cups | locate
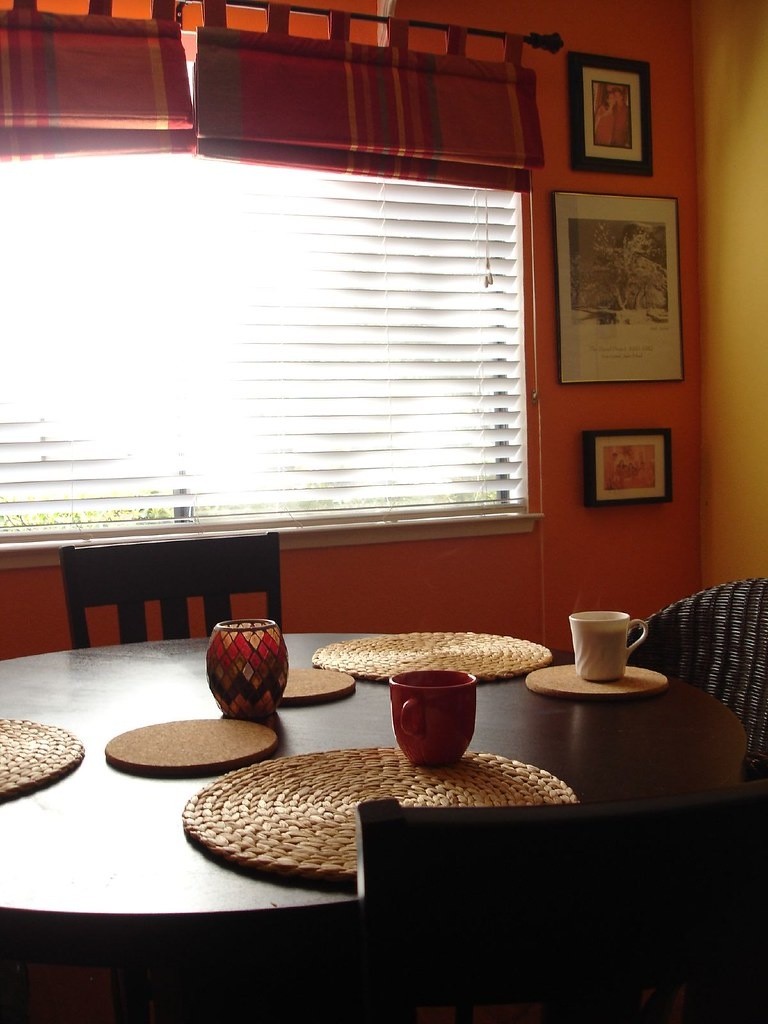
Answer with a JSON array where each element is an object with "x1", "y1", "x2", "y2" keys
[
  {"x1": 570, "y1": 612, "x2": 648, "y2": 682},
  {"x1": 388, "y1": 669, "x2": 478, "y2": 766}
]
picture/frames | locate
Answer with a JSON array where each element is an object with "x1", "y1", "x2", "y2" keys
[
  {"x1": 567, "y1": 50, "x2": 653, "y2": 176},
  {"x1": 582, "y1": 428, "x2": 673, "y2": 509},
  {"x1": 551, "y1": 190, "x2": 685, "y2": 384}
]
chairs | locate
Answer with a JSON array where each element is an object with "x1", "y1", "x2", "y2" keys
[
  {"x1": 356, "y1": 777, "x2": 768, "y2": 1024},
  {"x1": 625, "y1": 576, "x2": 768, "y2": 783},
  {"x1": 58, "y1": 531, "x2": 284, "y2": 650}
]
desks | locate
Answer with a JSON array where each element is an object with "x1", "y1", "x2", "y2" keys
[{"x1": 0, "y1": 631, "x2": 747, "y2": 1024}]
